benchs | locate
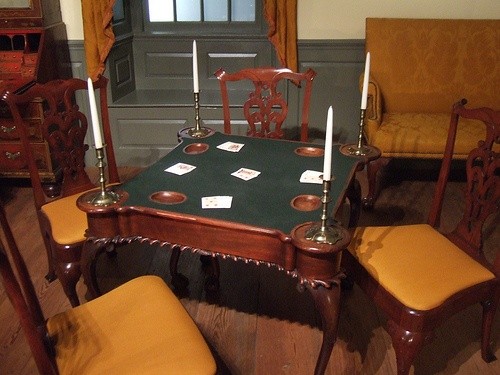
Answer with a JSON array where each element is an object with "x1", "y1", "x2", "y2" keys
[{"x1": 356, "y1": 17, "x2": 500, "y2": 208}]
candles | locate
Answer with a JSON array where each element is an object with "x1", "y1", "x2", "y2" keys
[
  {"x1": 359, "y1": 51, "x2": 370, "y2": 110},
  {"x1": 192, "y1": 39, "x2": 199, "y2": 92},
  {"x1": 87, "y1": 78, "x2": 102, "y2": 148},
  {"x1": 324, "y1": 104, "x2": 334, "y2": 180}
]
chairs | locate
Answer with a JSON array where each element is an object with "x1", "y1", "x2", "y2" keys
[
  {"x1": 0, "y1": 198, "x2": 228, "y2": 375},
  {"x1": 199, "y1": 67, "x2": 315, "y2": 265},
  {"x1": 314, "y1": 99, "x2": 500, "y2": 375},
  {"x1": 0, "y1": 74, "x2": 120, "y2": 308}
]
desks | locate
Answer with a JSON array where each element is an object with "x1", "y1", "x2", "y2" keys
[{"x1": 76, "y1": 128, "x2": 383, "y2": 375}]
